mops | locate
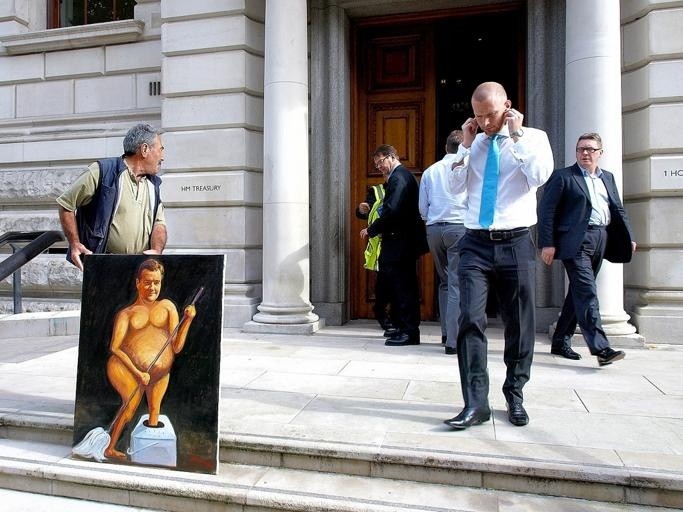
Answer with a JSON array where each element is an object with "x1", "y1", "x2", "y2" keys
[{"x1": 72, "y1": 285, "x2": 206, "y2": 463}]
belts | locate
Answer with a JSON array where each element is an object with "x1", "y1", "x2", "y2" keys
[
  {"x1": 427, "y1": 221, "x2": 464, "y2": 226},
  {"x1": 467, "y1": 228, "x2": 530, "y2": 242}
]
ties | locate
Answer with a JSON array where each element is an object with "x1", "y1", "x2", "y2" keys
[{"x1": 479, "y1": 134, "x2": 501, "y2": 230}]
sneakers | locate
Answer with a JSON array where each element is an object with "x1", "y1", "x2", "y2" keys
[{"x1": 441, "y1": 335, "x2": 458, "y2": 354}]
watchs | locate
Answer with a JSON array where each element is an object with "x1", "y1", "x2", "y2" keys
[{"x1": 510, "y1": 128, "x2": 524, "y2": 138}]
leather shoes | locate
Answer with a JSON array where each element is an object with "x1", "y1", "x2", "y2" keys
[
  {"x1": 384, "y1": 327, "x2": 420, "y2": 346},
  {"x1": 597, "y1": 347, "x2": 626, "y2": 366},
  {"x1": 444, "y1": 406, "x2": 491, "y2": 430},
  {"x1": 505, "y1": 401, "x2": 530, "y2": 427},
  {"x1": 550, "y1": 345, "x2": 582, "y2": 360}
]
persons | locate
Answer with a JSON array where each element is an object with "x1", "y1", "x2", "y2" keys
[
  {"x1": 537, "y1": 132, "x2": 636, "y2": 366},
  {"x1": 356, "y1": 172, "x2": 392, "y2": 329},
  {"x1": 360, "y1": 145, "x2": 430, "y2": 346},
  {"x1": 419, "y1": 129, "x2": 469, "y2": 354},
  {"x1": 443, "y1": 82, "x2": 554, "y2": 429},
  {"x1": 56, "y1": 124, "x2": 167, "y2": 271},
  {"x1": 104, "y1": 260, "x2": 196, "y2": 458}
]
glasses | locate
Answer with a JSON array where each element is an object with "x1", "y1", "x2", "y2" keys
[
  {"x1": 576, "y1": 147, "x2": 600, "y2": 153},
  {"x1": 374, "y1": 155, "x2": 390, "y2": 168}
]
